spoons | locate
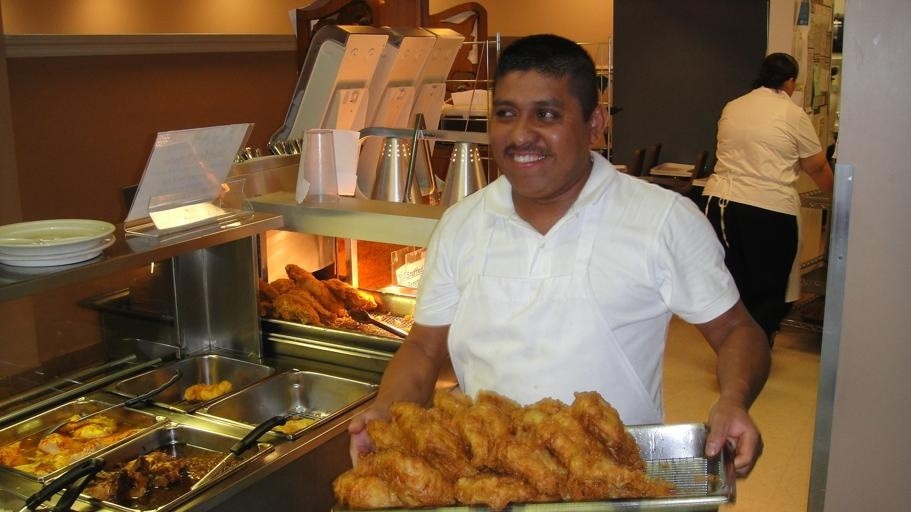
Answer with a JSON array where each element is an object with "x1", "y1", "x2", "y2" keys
[{"x1": 39, "y1": 370, "x2": 184, "y2": 444}]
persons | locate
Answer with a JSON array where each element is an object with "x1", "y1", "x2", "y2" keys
[
  {"x1": 701, "y1": 52, "x2": 835, "y2": 347},
  {"x1": 347, "y1": 34, "x2": 772, "y2": 480}
]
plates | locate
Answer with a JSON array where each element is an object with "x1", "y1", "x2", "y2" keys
[
  {"x1": 429, "y1": 423, "x2": 736, "y2": 511},
  {"x1": 650, "y1": 160, "x2": 697, "y2": 178},
  {"x1": 1, "y1": 221, "x2": 116, "y2": 269}
]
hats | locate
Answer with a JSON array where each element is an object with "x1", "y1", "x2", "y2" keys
[{"x1": 760, "y1": 53, "x2": 801, "y2": 82}]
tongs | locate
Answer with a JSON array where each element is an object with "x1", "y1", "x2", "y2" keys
[
  {"x1": 348, "y1": 305, "x2": 411, "y2": 340},
  {"x1": 402, "y1": 115, "x2": 440, "y2": 212}
]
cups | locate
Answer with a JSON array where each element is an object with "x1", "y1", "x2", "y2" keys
[{"x1": 295, "y1": 127, "x2": 339, "y2": 207}]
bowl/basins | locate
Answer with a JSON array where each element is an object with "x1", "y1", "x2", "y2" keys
[
  {"x1": 257, "y1": 286, "x2": 418, "y2": 353},
  {"x1": 195, "y1": 368, "x2": 381, "y2": 442},
  {"x1": 43, "y1": 420, "x2": 275, "y2": 512},
  {"x1": 0, "y1": 395, "x2": 170, "y2": 484},
  {"x1": 103, "y1": 353, "x2": 275, "y2": 415}
]
